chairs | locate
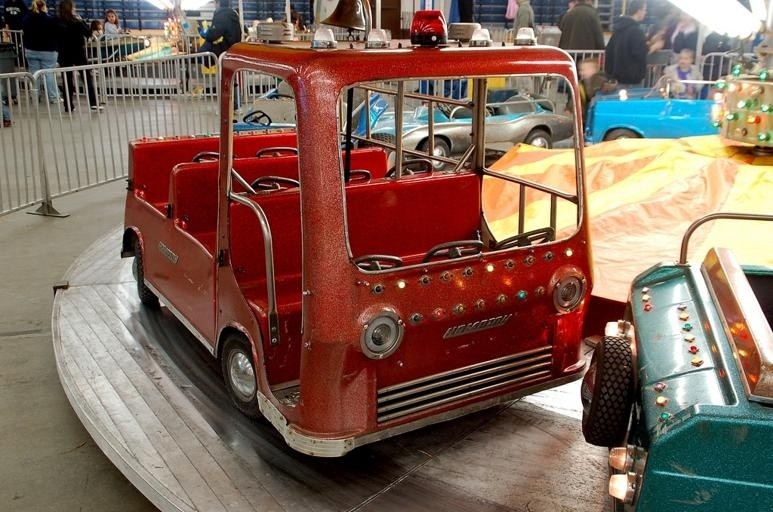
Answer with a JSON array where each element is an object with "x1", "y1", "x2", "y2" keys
[
  {"x1": 494, "y1": 95, "x2": 533, "y2": 116},
  {"x1": 441, "y1": 99, "x2": 489, "y2": 125}
]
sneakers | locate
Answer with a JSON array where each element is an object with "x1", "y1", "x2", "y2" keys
[
  {"x1": 51, "y1": 97, "x2": 65, "y2": 103},
  {"x1": 90, "y1": 104, "x2": 105, "y2": 111}
]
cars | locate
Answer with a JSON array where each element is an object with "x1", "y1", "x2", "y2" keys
[
  {"x1": 227, "y1": 81, "x2": 306, "y2": 129},
  {"x1": 586, "y1": 76, "x2": 716, "y2": 142},
  {"x1": 368, "y1": 89, "x2": 575, "y2": 172}
]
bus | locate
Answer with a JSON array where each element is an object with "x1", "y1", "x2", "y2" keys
[{"x1": 120, "y1": 8, "x2": 598, "y2": 462}]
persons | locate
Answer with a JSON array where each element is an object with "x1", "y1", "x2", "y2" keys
[
  {"x1": 102, "y1": 8, "x2": 124, "y2": 37},
  {"x1": 87, "y1": 20, "x2": 105, "y2": 42},
  {"x1": 662, "y1": 46, "x2": 710, "y2": 101},
  {"x1": 564, "y1": 60, "x2": 612, "y2": 126},
  {"x1": 505, "y1": 0, "x2": 772, "y2": 87},
  {"x1": 21, "y1": 0, "x2": 60, "y2": 106},
  {"x1": 193, "y1": 0, "x2": 241, "y2": 116},
  {"x1": 3, "y1": 0, "x2": 29, "y2": 68},
  {"x1": 0, "y1": 25, "x2": 16, "y2": 129},
  {"x1": 53, "y1": 1, "x2": 102, "y2": 112},
  {"x1": 284, "y1": 4, "x2": 302, "y2": 22}
]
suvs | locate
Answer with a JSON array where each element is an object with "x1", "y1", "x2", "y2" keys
[{"x1": 581, "y1": 206, "x2": 772, "y2": 510}]
